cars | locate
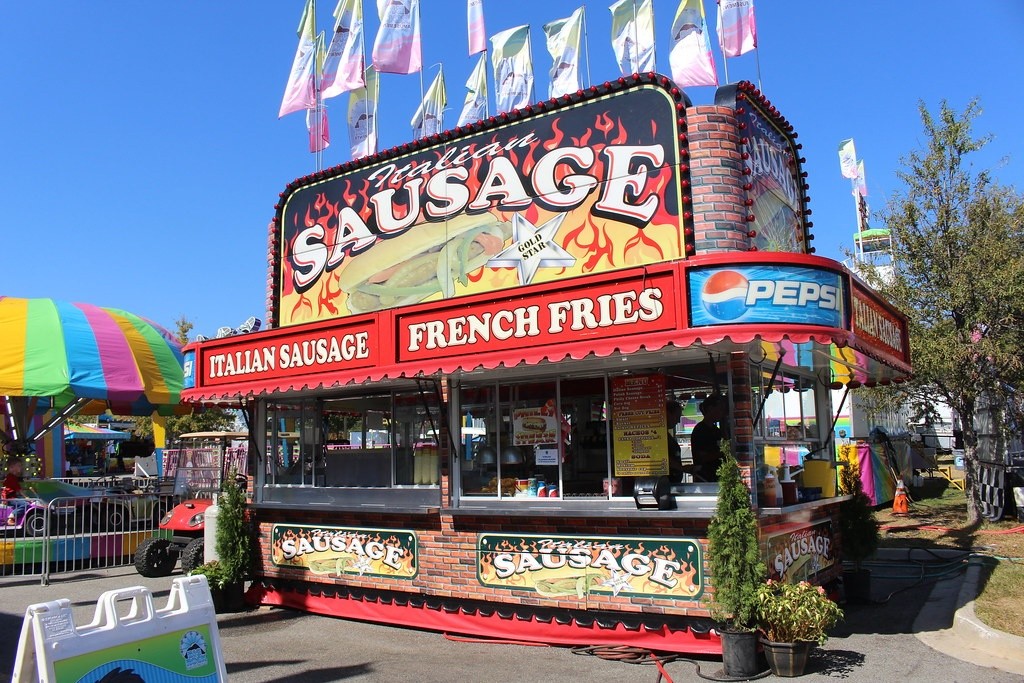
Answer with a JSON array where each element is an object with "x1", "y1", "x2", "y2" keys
[
  {"x1": 87, "y1": 482, "x2": 166, "y2": 532},
  {"x1": 0, "y1": 486, "x2": 55, "y2": 537}
]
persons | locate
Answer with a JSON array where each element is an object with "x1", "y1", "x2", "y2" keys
[
  {"x1": 666, "y1": 400, "x2": 684, "y2": 484},
  {"x1": 691, "y1": 392, "x2": 730, "y2": 482},
  {"x1": 1, "y1": 458, "x2": 31, "y2": 526}
]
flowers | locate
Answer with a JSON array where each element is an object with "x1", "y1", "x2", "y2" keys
[{"x1": 756, "y1": 574, "x2": 845, "y2": 644}]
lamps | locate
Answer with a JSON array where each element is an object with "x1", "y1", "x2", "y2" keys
[{"x1": 476, "y1": 384, "x2": 527, "y2": 468}]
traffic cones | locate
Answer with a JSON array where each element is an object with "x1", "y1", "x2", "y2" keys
[{"x1": 888, "y1": 472, "x2": 910, "y2": 518}]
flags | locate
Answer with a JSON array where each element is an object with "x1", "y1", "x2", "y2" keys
[
  {"x1": 277, "y1": 0, "x2": 758, "y2": 162},
  {"x1": 838, "y1": 138, "x2": 866, "y2": 196}
]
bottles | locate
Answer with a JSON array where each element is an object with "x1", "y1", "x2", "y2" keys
[
  {"x1": 765, "y1": 464, "x2": 776, "y2": 507},
  {"x1": 770, "y1": 420, "x2": 785, "y2": 437}
]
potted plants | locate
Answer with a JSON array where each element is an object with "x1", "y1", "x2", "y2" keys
[
  {"x1": 701, "y1": 434, "x2": 771, "y2": 677},
  {"x1": 835, "y1": 440, "x2": 881, "y2": 606},
  {"x1": 187, "y1": 461, "x2": 249, "y2": 613}
]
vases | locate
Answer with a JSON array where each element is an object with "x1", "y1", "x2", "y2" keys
[{"x1": 761, "y1": 635, "x2": 812, "y2": 676}]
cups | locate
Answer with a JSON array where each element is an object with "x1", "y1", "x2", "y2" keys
[
  {"x1": 528, "y1": 478, "x2": 537, "y2": 496},
  {"x1": 538, "y1": 481, "x2": 546, "y2": 497},
  {"x1": 549, "y1": 485, "x2": 557, "y2": 497}
]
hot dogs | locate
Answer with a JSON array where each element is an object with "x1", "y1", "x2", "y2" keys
[
  {"x1": 522, "y1": 417, "x2": 547, "y2": 432},
  {"x1": 788, "y1": 554, "x2": 812, "y2": 582}
]
